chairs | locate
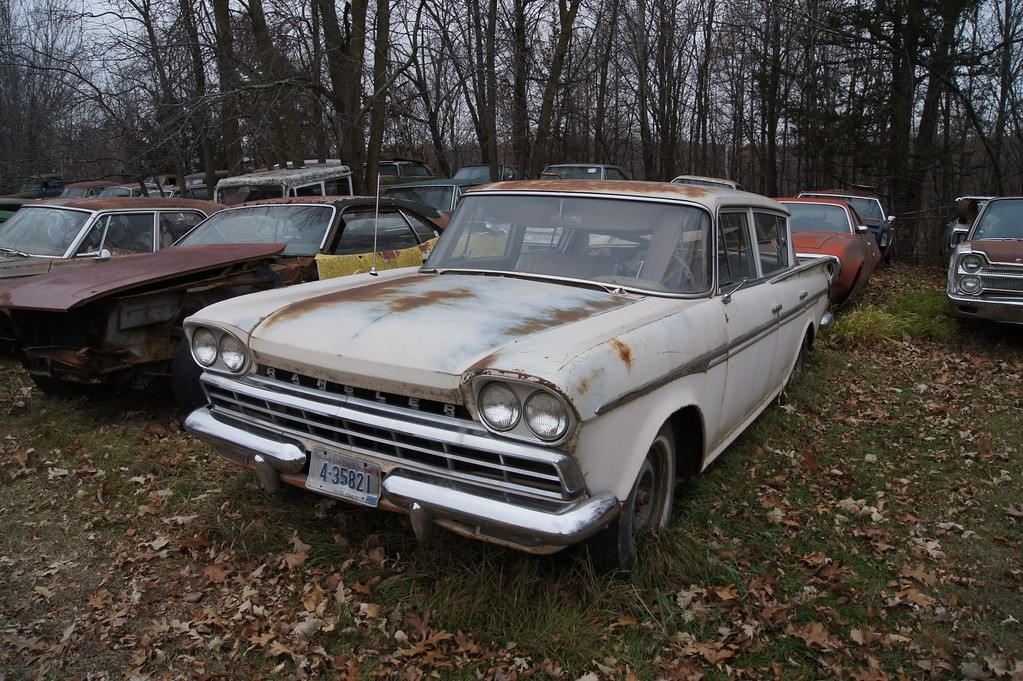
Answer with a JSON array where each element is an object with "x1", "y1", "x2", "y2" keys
[
  {"x1": 291, "y1": 220, "x2": 420, "y2": 254},
  {"x1": 521, "y1": 237, "x2": 765, "y2": 294},
  {"x1": 92, "y1": 222, "x2": 187, "y2": 251}
]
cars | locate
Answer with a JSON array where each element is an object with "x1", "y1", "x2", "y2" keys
[
  {"x1": 1, "y1": 198, "x2": 245, "y2": 345},
  {"x1": 771, "y1": 198, "x2": 881, "y2": 307},
  {"x1": 942, "y1": 197, "x2": 995, "y2": 265},
  {"x1": 793, "y1": 191, "x2": 895, "y2": 266},
  {"x1": 1, "y1": 157, "x2": 747, "y2": 247},
  {"x1": 1, "y1": 196, "x2": 530, "y2": 414},
  {"x1": 946, "y1": 196, "x2": 1022, "y2": 330}
]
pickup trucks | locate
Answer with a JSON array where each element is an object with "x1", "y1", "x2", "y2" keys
[{"x1": 182, "y1": 177, "x2": 842, "y2": 581}]
